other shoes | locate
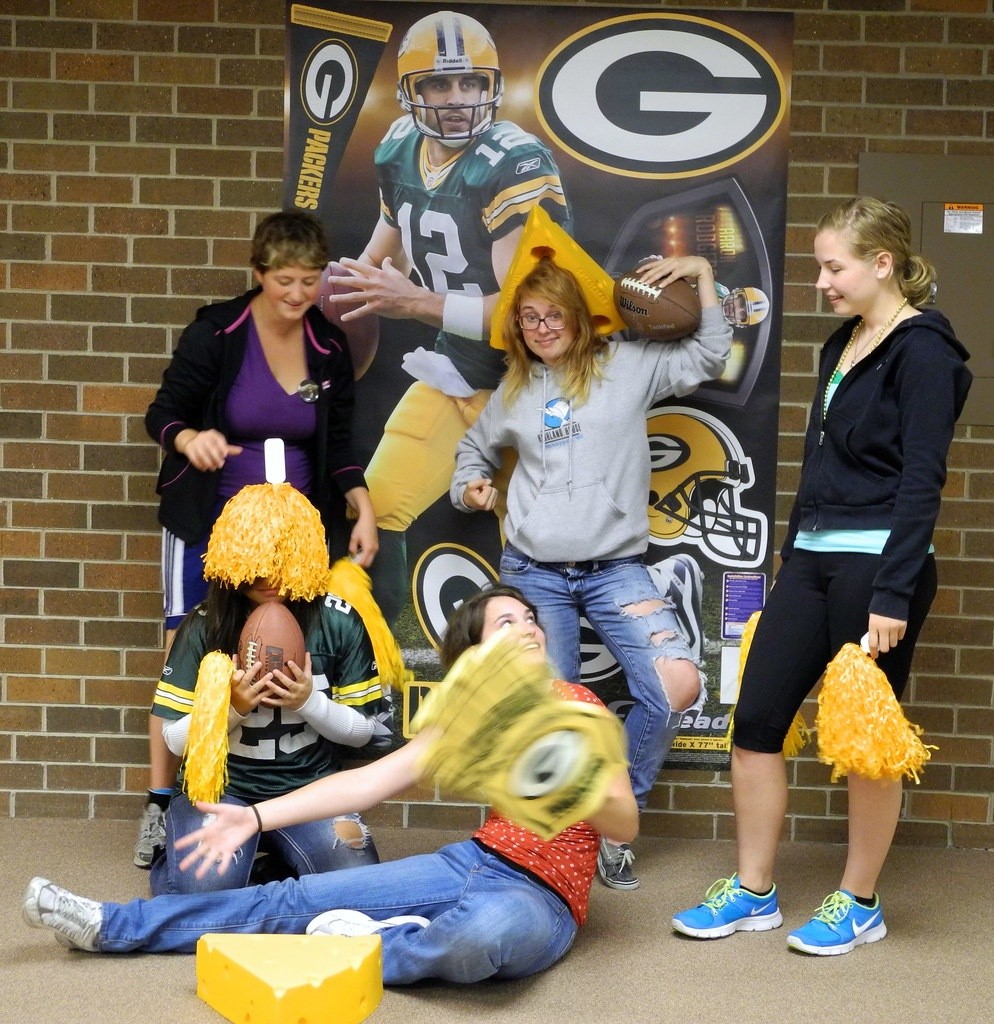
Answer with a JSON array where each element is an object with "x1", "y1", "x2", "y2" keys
[{"x1": 652, "y1": 554, "x2": 710, "y2": 670}]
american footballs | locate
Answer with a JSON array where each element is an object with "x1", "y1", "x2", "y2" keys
[
  {"x1": 237, "y1": 598, "x2": 305, "y2": 704},
  {"x1": 316, "y1": 264, "x2": 380, "y2": 356},
  {"x1": 612, "y1": 270, "x2": 702, "y2": 343}
]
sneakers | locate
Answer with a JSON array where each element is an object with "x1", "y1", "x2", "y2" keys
[
  {"x1": 787, "y1": 890, "x2": 887, "y2": 955},
  {"x1": 22, "y1": 877, "x2": 102, "y2": 951},
  {"x1": 133, "y1": 803, "x2": 167, "y2": 867},
  {"x1": 673, "y1": 871, "x2": 783, "y2": 939},
  {"x1": 306, "y1": 909, "x2": 431, "y2": 938},
  {"x1": 597, "y1": 840, "x2": 639, "y2": 890}
]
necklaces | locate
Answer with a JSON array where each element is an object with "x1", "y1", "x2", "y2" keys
[{"x1": 822, "y1": 296, "x2": 909, "y2": 420}]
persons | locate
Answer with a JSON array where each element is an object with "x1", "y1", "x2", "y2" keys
[
  {"x1": 133, "y1": 209, "x2": 381, "y2": 867},
  {"x1": 595, "y1": 250, "x2": 772, "y2": 345},
  {"x1": 447, "y1": 252, "x2": 736, "y2": 888},
  {"x1": 21, "y1": 579, "x2": 639, "y2": 986},
  {"x1": 328, "y1": 10, "x2": 710, "y2": 667},
  {"x1": 672, "y1": 195, "x2": 972, "y2": 953},
  {"x1": 146, "y1": 479, "x2": 393, "y2": 901}
]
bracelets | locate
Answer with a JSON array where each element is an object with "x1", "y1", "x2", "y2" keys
[
  {"x1": 441, "y1": 292, "x2": 485, "y2": 342},
  {"x1": 250, "y1": 804, "x2": 263, "y2": 834}
]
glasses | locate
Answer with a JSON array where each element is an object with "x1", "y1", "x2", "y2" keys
[{"x1": 516, "y1": 313, "x2": 567, "y2": 329}]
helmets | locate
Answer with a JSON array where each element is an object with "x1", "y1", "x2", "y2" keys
[
  {"x1": 396, "y1": 11, "x2": 504, "y2": 127},
  {"x1": 721, "y1": 286, "x2": 770, "y2": 326}
]
